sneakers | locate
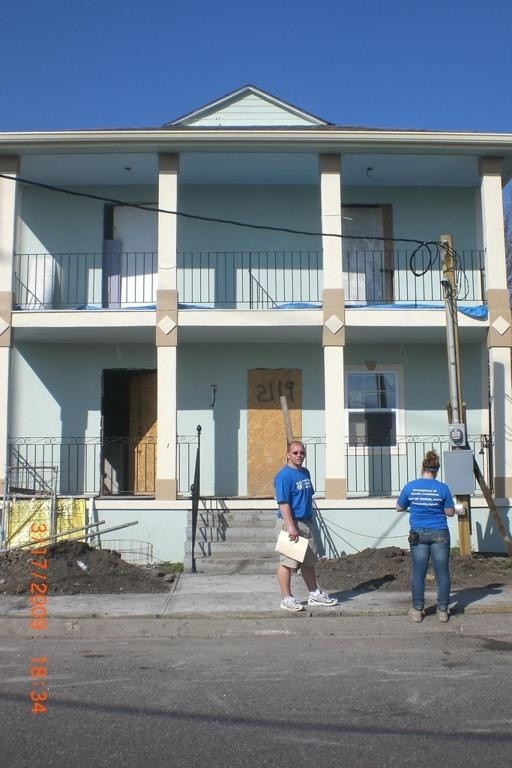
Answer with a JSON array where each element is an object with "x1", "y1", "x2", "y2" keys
[
  {"x1": 410, "y1": 607, "x2": 423, "y2": 622},
  {"x1": 307, "y1": 592, "x2": 338, "y2": 606},
  {"x1": 280, "y1": 596, "x2": 305, "y2": 612},
  {"x1": 436, "y1": 609, "x2": 449, "y2": 623}
]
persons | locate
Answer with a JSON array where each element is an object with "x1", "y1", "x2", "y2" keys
[
  {"x1": 273, "y1": 441, "x2": 338, "y2": 612},
  {"x1": 395, "y1": 450, "x2": 455, "y2": 623}
]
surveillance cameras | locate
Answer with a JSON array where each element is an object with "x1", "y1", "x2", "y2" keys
[{"x1": 440, "y1": 275, "x2": 448, "y2": 286}]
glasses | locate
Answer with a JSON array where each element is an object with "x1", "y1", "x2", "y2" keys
[{"x1": 292, "y1": 450, "x2": 305, "y2": 455}]
